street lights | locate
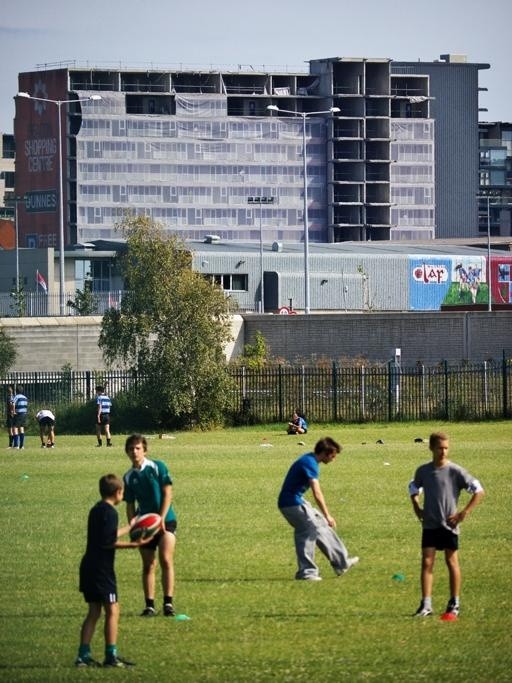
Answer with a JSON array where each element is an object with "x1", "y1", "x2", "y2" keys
[
  {"x1": 247, "y1": 194, "x2": 274, "y2": 314},
  {"x1": 475, "y1": 189, "x2": 501, "y2": 311},
  {"x1": 266, "y1": 103, "x2": 342, "y2": 314},
  {"x1": 12, "y1": 91, "x2": 101, "y2": 313},
  {"x1": 3, "y1": 193, "x2": 30, "y2": 316}
]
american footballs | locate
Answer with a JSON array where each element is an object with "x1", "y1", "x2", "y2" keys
[{"x1": 130, "y1": 512, "x2": 162, "y2": 542}]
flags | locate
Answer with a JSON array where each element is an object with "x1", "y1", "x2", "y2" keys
[{"x1": 32, "y1": 270, "x2": 48, "y2": 295}]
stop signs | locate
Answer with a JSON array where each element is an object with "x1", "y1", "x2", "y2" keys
[{"x1": 278, "y1": 306, "x2": 289, "y2": 315}]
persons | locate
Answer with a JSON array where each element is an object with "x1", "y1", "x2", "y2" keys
[
  {"x1": 35, "y1": 409, "x2": 57, "y2": 448},
  {"x1": 13, "y1": 384, "x2": 29, "y2": 450},
  {"x1": 277, "y1": 435, "x2": 362, "y2": 582},
  {"x1": 285, "y1": 412, "x2": 309, "y2": 434},
  {"x1": 5, "y1": 394, "x2": 16, "y2": 448},
  {"x1": 119, "y1": 431, "x2": 179, "y2": 618},
  {"x1": 406, "y1": 431, "x2": 486, "y2": 622},
  {"x1": 94, "y1": 385, "x2": 113, "y2": 445},
  {"x1": 74, "y1": 471, "x2": 155, "y2": 668}
]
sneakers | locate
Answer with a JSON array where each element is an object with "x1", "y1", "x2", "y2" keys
[
  {"x1": 51, "y1": 445, "x2": 54, "y2": 449},
  {"x1": 6, "y1": 447, "x2": 12, "y2": 449},
  {"x1": 335, "y1": 556, "x2": 359, "y2": 577},
  {"x1": 444, "y1": 607, "x2": 459, "y2": 616},
  {"x1": 20, "y1": 446, "x2": 24, "y2": 450},
  {"x1": 106, "y1": 443, "x2": 112, "y2": 447},
  {"x1": 103, "y1": 656, "x2": 136, "y2": 669},
  {"x1": 164, "y1": 603, "x2": 174, "y2": 615},
  {"x1": 75, "y1": 656, "x2": 101, "y2": 669},
  {"x1": 13, "y1": 447, "x2": 19, "y2": 449},
  {"x1": 144, "y1": 606, "x2": 155, "y2": 617},
  {"x1": 412, "y1": 608, "x2": 434, "y2": 617},
  {"x1": 41, "y1": 445, "x2": 46, "y2": 448},
  {"x1": 95, "y1": 444, "x2": 102, "y2": 447},
  {"x1": 298, "y1": 575, "x2": 322, "y2": 581}
]
